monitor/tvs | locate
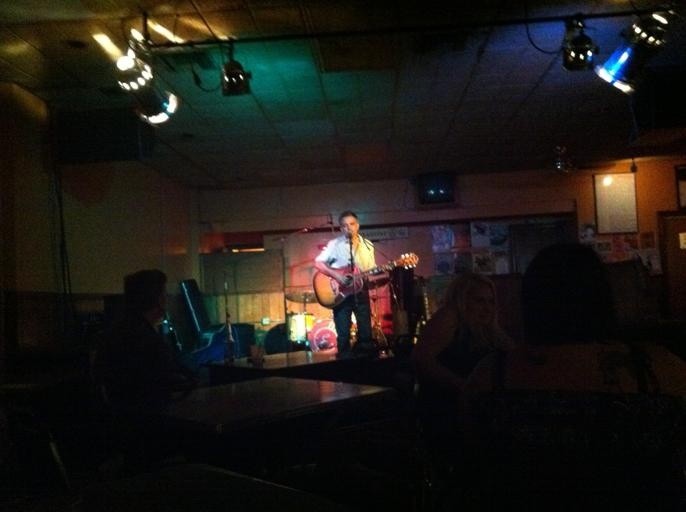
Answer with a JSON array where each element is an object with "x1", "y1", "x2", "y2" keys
[{"x1": 415, "y1": 169, "x2": 459, "y2": 209}]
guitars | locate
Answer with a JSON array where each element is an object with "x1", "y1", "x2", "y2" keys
[{"x1": 313, "y1": 253, "x2": 420, "y2": 309}]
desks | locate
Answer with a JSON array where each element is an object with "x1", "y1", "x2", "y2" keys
[
  {"x1": 229, "y1": 348, "x2": 365, "y2": 372},
  {"x1": 140, "y1": 375, "x2": 403, "y2": 429}
]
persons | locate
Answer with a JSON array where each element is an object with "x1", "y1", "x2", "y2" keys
[
  {"x1": 313, "y1": 209, "x2": 390, "y2": 359},
  {"x1": 454, "y1": 240, "x2": 686, "y2": 439},
  {"x1": 414, "y1": 270, "x2": 515, "y2": 457},
  {"x1": 92, "y1": 267, "x2": 196, "y2": 399}
]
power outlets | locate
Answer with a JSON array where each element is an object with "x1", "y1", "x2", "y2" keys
[{"x1": 216, "y1": 37, "x2": 254, "y2": 100}]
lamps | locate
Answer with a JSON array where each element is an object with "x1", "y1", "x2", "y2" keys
[
  {"x1": 594, "y1": 4, "x2": 678, "y2": 99},
  {"x1": 115, "y1": 36, "x2": 180, "y2": 127},
  {"x1": 562, "y1": 14, "x2": 600, "y2": 71}
]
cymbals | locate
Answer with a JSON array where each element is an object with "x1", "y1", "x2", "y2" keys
[
  {"x1": 369, "y1": 294, "x2": 386, "y2": 301},
  {"x1": 366, "y1": 278, "x2": 390, "y2": 290},
  {"x1": 284, "y1": 289, "x2": 317, "y2": 303}
]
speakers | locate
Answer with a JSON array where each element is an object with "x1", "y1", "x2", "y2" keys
[{"x1": 55, "y1": 107, "x2": 151, "y2": 164}]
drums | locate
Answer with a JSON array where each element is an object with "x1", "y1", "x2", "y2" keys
[
  {"x1": 307, "y1": 317, "x2": 339, "y2": 353},
  {"x1": 286, "y1": 312, "x2": 314, "y2": 343}
]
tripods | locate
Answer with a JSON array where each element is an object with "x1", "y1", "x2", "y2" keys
[{"x1": 371, "y1": 287, "x2": 395, "y2": 357}]
